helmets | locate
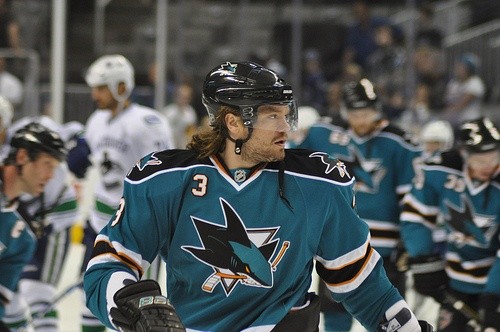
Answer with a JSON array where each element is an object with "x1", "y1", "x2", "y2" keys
[
  {"x1": 84, "y1": 54, "x2": 135, "y2": 100},
  {"x1": 202, "y1": 60, "x2": 294, "y2": 126},
  {"x1": 341, "y1": 79, "x2": 379, "y2": 113},
  {"x1": 454, "y1": 115, "x2": 500, "y2": 152},
  {"x1": 10, "y1": 122, "x2": 68, "y2": 160},
  {"x1": 421, "y1": 121, "x2": 454, "y2": 149}
]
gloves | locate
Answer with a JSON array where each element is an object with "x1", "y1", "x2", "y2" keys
[
  {"x1": 407, "y1": 252, "x2": 450, "y2": 303},
  {"x1": 64, "y1": 134, "x2": 110, "y2": 178},
  {"x1": 110, "y1": 279, "x2": 186, "y2": 332}
]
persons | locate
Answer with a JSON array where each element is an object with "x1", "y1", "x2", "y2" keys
[
  {"x1": 284, "y1": 77, "x2": 500, "y2": 332},
  {"x1": 83, "y1": 54, "x2": 177, "y2": 332},
  {"x1": 163, "y1": 83, "x2": 197, "y2": 145},
  {"x1": 84, "y1": 61, "x2": 433, "y2": 332},
  {"x1": 303, "y1": 0, "x2": 484, "y2": 159},
  {"x1": 0, "y1": 71, "x2": 85, "y2": 332}
]
